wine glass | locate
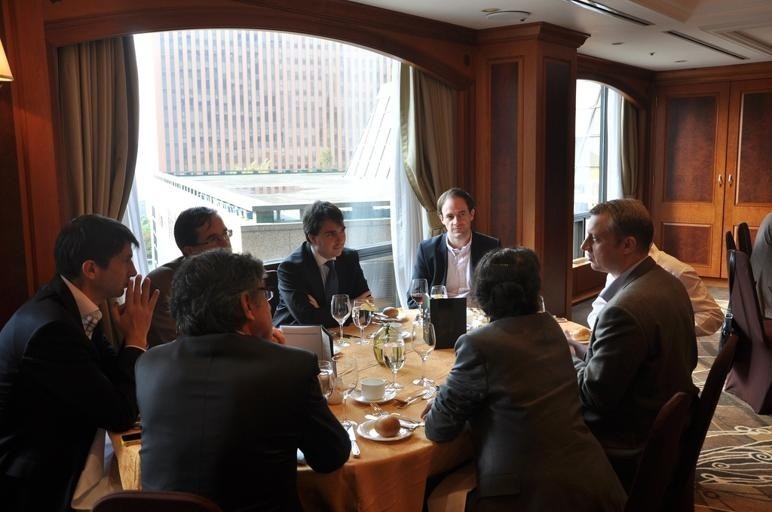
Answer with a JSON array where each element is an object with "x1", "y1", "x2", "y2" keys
[{"x1": 317, "y1": 278, "x2": 449, "y2": 404}]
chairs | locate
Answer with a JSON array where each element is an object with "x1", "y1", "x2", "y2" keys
[
  {"x1": 734, "y1": 222, "x2": 753, "y2": 259},
  {"x1": 720, "y1": 249, "x2": 771, "y2": 415},
  {"x1": 723, "y1": 229, "x2": 734, "y2": 274},
  {"x1": 92, "y1": 488, "x2": 219, "y2": 512},
  {"x1": 622, "y1": 387, "x2": 695, "y2": 511},
  {"x1": 693, "y1": 330, "x2": 736, "y2": 463}
]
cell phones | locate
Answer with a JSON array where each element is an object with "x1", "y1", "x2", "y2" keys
[{"x1": 119, "y1": 432, "x2": 142, "y2": 447}]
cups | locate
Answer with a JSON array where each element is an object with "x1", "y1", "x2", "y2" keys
[{"x1": 538, "y1": 294, "x2": 545, "y2": 313}]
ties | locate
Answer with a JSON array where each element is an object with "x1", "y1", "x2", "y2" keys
[{"x1": 325, "y1": 260, "x2": 339, "y2": 305}]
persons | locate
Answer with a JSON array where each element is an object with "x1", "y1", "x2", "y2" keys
[
  {"x1": 749, "y1": 212, "x2": 772, "y2": 318},
  {"x1": 142, "y1": 207, "x2": 230, "y2": 347},
  {"x1": 568, "y1": 198, "x2": 698, "y2": 446},
  {"x1": 273, "y1": 202, "x2": 375, "y2": 328},
  {"x1": 587, "y1": 244, "x2": 725, "y2": 338},
  {"x1": 408, "y1": 186, "x2": 500, "y2": 309},
  {"x1": 0, "y1": 213, "x2": 161, "y2": 512},
  {"x1": 134, "y1": 248, "x2": 351, "y2": 512},
  {"x1": 424, "y1": 246, "x2": 630, "y2": 512}
]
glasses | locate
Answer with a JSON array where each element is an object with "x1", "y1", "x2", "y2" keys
[
  {"x1": 232, "y1": 284, "x2": 274, "y2": 304},
  {"x1": 189, "y1": 229, "x2": 233, "y2": 249}
]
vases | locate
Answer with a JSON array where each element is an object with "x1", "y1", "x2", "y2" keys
[{"x1": 372, "y1": 323, "x2": 406, "y2": 369}]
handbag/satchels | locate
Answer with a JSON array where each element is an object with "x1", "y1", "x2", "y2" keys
[{"x1": 718, "y1": 321, "x2": 732, "y2": 352}]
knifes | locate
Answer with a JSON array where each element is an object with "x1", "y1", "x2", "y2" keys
[{"x1": 348, "y1": 424, "x2": 361, "y2": 458}]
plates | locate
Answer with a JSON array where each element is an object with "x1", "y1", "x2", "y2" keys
[
  {"x1": 357, "y1": 419, "x2": 412, "y2": 442},
  {"x1": 572, "y1": 328, "x2": 591, "y2": 345}
]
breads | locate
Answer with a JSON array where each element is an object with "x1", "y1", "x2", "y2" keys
[
  {"x1": 574, "y1": 328, "x2": 590, "y2": 341},
  {"x1": 383, "y1": 307, "x2": 398, "y2": 317},
  {"x1": 374, "y1": 415, "x2": 400, "y2": 438}
]
